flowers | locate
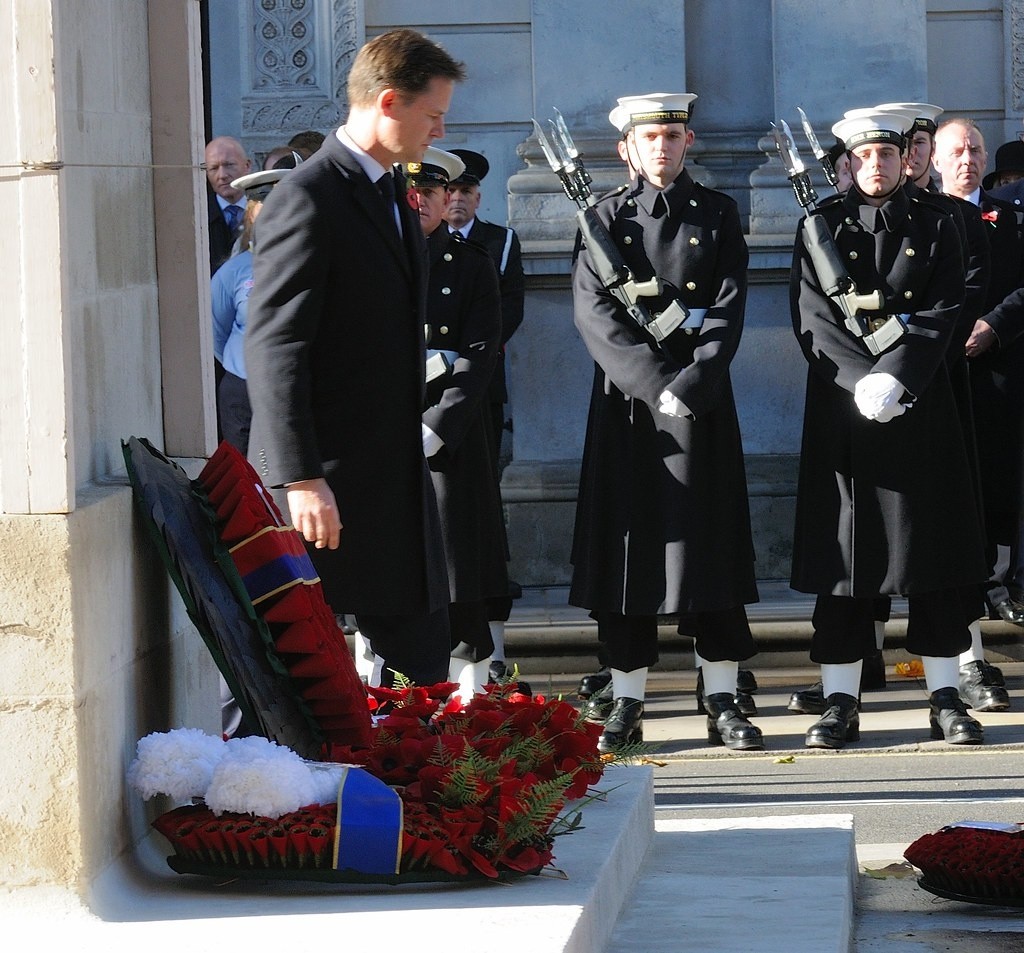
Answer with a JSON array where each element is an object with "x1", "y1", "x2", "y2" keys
[
  {"x1": 980, "y1": 210, "x2": 999, "y2": 229},
  {"x1": 403, "y1": 176, "x2": 417, "y2": 211},
  {"x1": 904, "y1": 819, "x2": 1024, "y2": 909},
  {"x1": 200, "y1": 432, "x2": 374, "y2": 747},
  {"x1": 127, "y1": 672, "x2": 669, "y2": 892}
]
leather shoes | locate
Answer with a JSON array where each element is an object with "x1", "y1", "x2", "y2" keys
[
  {"x1": 696, "y1": 672, "x2": 757, "y2": 714},
  {"x1": 578, "y1": 667, "x2": 611, "y2": 698},
  {"x1": 586, "y1": 681, "x2": 613, "y2": 721},
  {"x1": 958, "y1": 660, "x2": 1010, "y2": 711},
  {"x1": 596, "y1": 696, "x2": 645, "y2": 752},
  {"x1": 804, "y1": 703, "x2": 860, "y2": 747},
  {"x1": 928, "y1": 686, "x2": 984, "y2": 742},
  {"x1": 989, "y1": 598, "x2": 1023, "y2": 623},
  {"x1": 983, "y1": 664, "x2": 1005, "y2": 687},
  {"x1": 488, "y1": 662, "x2": 532, "y2": 699},
  {"x1": 706, "y1": 704, "x2": 765, "y2": 750},
  {"x1": 737, "y1": 670, "x2": 757, "y2": 693},
  {"x1": 787, "y1": 680, "x2": 827, "y2": 714}
]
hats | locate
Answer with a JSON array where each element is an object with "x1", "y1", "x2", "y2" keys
[
  {"x1": 408, "y1": 144, "x2": 467, "y2": 190},
  {"x1": 230, "y1": 168, "x2": 296, "y2": 197},
  {"x1": 443, "y1": 148, "x2": 491, "y2": 185},
  {"x1": 606, "y1": 92, "x2": 945, "y2": 150}
]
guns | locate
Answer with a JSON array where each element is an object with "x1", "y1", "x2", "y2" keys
[
  {"x1": 530, "y1": 103, "x2": 691, "y2": 353},
  {"x1": 770, "y1": 107, "x2": 908, "y2": 358}
]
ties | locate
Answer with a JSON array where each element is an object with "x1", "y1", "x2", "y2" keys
[
  {"x1": 224, "y1": 204, "x2": 239, "y2": 236},
  {"x1": 379, "y1": 173, "x2": 398, "y2": 238}
]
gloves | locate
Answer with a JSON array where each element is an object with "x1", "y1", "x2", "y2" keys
[
  {"x1": 658, "y1": 390, "x2": 692, "y2": 417},
  {"x1": 421, "y1": 423, "x2": 444, "y2": 457},
  {"x1": 854, "y1": 373, "x2": 907, "y2": 423}
]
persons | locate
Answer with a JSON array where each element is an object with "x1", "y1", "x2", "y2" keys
[
  {"x1": 243, "y1": 28, "x2": 469, "y2": 710},
  {"x1": 207, "y1": 90, "x2": 1024, "y2": 750}
]
